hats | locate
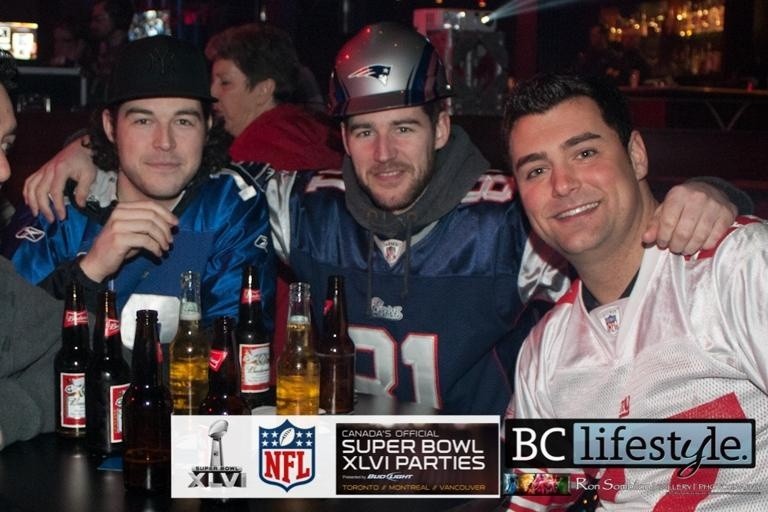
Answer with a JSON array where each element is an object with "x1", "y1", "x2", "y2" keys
[{"x1": 109, "y1": 35, "x2": 210, "y2": 101}]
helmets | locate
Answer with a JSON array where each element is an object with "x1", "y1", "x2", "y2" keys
[{"x1": 329, "y1": 20, "x2": 454, "y2": 117}]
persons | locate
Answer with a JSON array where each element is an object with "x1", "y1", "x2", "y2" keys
[
  {"x1": 21, "y1": 21, "x2": 755, "y2": 420},
  {"x1": 500, "y1": 72, "x2": 764, "y2": 512},
  {"x1": 206, "y1": 22, "x2": 344, "y2": 171},
  {"x1": 0, "y1": 47, "x2": 97, "y2": 448},
  {"x1": 9, "y1": 36, "x2": 276, "y2": 383}
]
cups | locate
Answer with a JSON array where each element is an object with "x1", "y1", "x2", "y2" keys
[{"x1": 629, "y1": 70, "x2": 641, "y2": 88}]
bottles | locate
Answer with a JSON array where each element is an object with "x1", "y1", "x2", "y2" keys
[
  {"x1": 669, "y1": 42, "x2": 720, "y2": 75},
  {"x1": 235, "y1": 264, "x2": 275, "y2": 408},
  {"x1": 198, "y1": 315, "x2": 251, "y2": 415},
  {"x1": 609, "y1": 0, "x2": 725, "y2": 43},
  {"x1": 122, "y1": 311, "x2": 174, "y2": 490},
  {"x1": 86, "y1": 291, "x2": 131, "y2": 458},
  {"x1": 53, "y1": 279, "x2": 93, "y2": 437},
  {"x1": 315, "y1": 274, "x2": 355, "y2": 415},
  {"x1": 276, "y1": 282, "x2": 321, "y2": 415},
  {"x1": 168, "y1": 271, "x2": 211, "y2": 415}
]
